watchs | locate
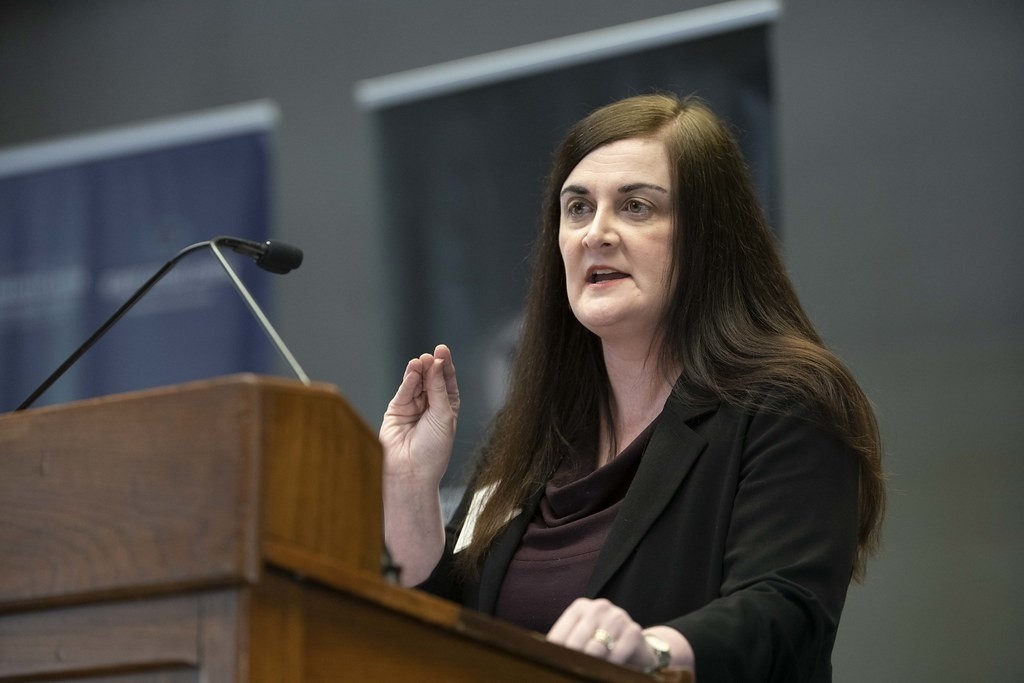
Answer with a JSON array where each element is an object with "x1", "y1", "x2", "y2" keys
[{"x1": 641, "y1": 630, "x2": 672, "y2": 675}]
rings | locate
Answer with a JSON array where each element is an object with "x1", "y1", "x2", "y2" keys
[{"x1": 592, "y1": 628, "x2": 616, "y2": 652}]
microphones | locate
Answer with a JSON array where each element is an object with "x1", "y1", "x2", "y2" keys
[{"x1": 12, "y1": 239, "x2": 312, "y2": 416}]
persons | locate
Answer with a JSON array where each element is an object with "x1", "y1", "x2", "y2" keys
[{"x1": 377, "y1": 95, "x2": 891, "y2": 683}]
